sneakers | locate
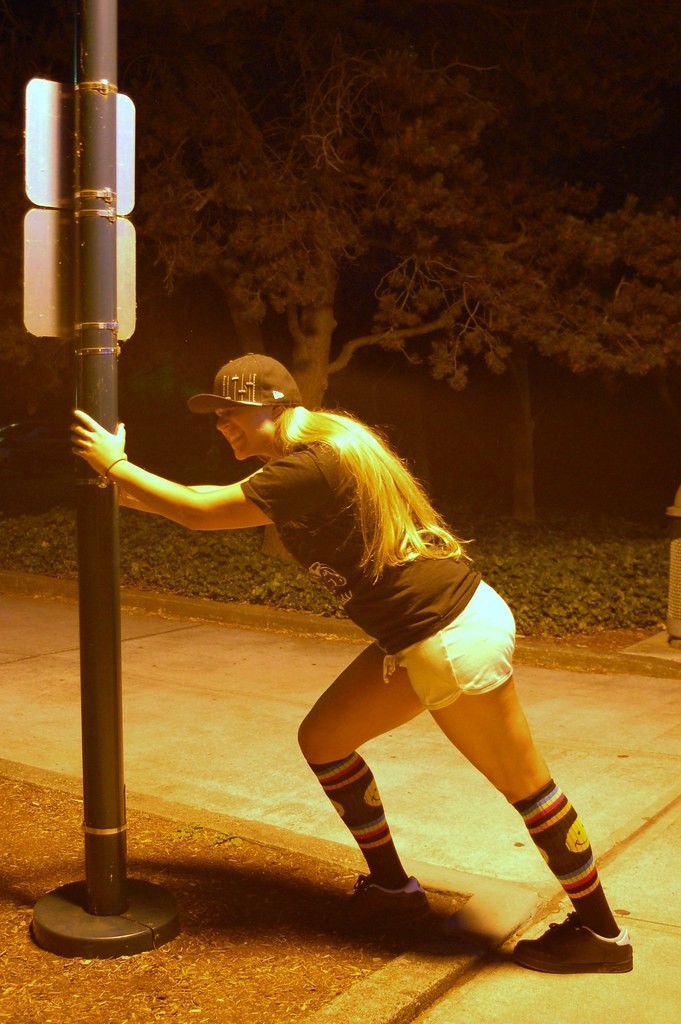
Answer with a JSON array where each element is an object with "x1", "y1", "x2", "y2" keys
[
  {"x1": 336, "y1": 875, "x2": 433, "y2": 926},
  {"x1": 509, "y1": 915, "x2": 636, "y2": 975}
]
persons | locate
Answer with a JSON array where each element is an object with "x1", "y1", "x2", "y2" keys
[{"x1": 76, "y1": 355, "x2": 633, "y2": 973}]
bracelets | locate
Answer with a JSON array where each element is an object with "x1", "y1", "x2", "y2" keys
[{"x1": 106, "y1": 458, "x2": 127, "y2": 479}]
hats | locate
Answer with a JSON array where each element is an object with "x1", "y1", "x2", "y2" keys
[{"x1": 185, "y1": 352, "x2": 304, "y2": 415}]
